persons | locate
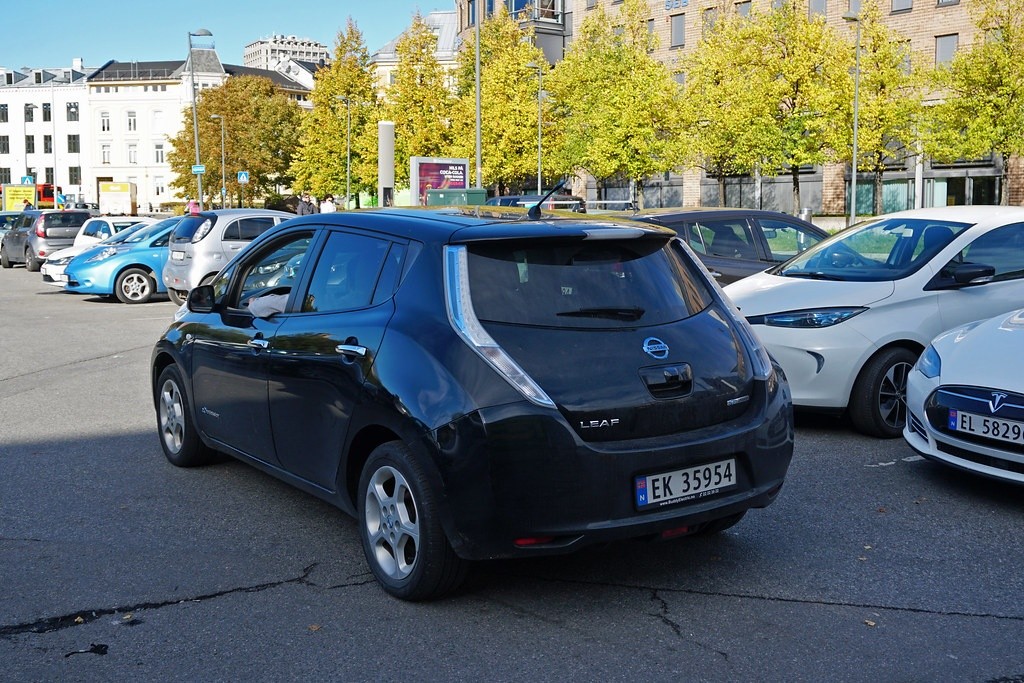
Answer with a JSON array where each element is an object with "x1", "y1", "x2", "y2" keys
[
  {"x1": 23, "y1": 199, "x2": 36, "y2": 210},
  {"x1": 183, "y1": 198, "x2": 201, "y2": 213},
  {"x1": 320, "y1": 194, "x2": 337, "y2": 213},
  {"x1": 248, "y1": 293, "x2": 289, "y2": 319},
  {"x1": 297, "y1": 193, "x2": 318, "y2": 216}
]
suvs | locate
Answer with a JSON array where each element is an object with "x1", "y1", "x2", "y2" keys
[
  {"x1": 150, "y1": 210, "x2": 795, "y2": 603},
  {"x1": 622, "y1": 206, "x2": 1024, "y2": 488}
]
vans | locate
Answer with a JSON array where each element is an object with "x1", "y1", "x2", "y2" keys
[
  {"x1": 0, "y1": 209, "x2": 303, "y2": 306},
  {"x1": 482, "y1": 195, "x2": 586, "y2": 211}
]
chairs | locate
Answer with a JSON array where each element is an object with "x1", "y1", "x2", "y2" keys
[
  {"x1": 345, "y1": 246, "x2": 399, "y2": 299},
  {"x1": 473, "y1": 249, "x2": 521, "y2": 290},
  {"x1": 914, "y1": 226, "x2": 954, "y2": 268},
  {"x1": 709, "y1": 226, "x2": 748, "y2": 255}
]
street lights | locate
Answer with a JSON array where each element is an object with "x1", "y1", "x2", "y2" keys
[
  {"x1": 23, "y1": 102, "x2": 39, "y2": 178},
  {"x1": 840, "y1": 11, "x2": 860, "y2": 229},
  {"x1": 208, "y1": 114, "x2": 227, "y2": 211},
  {"x1": 336, "y1": 94, "x2": 350, "y2": 208},
  {"x1": 186, "y1": 27, "x2": 214, "y2": 215},
  {"x1": 524, "y1": 62, "x2": 542, "y2": 201}
]
